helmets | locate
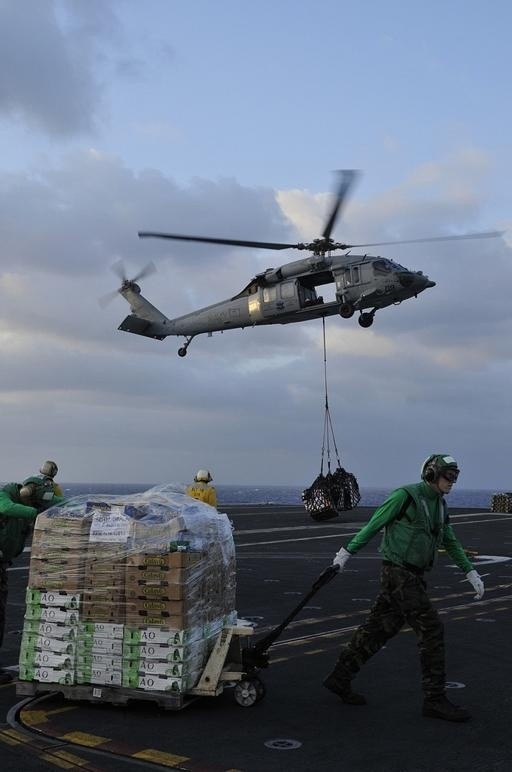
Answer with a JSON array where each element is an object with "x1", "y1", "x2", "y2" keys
[
  {"x1": 421, "y1": 452, "x2": 458, "y2": 484},
  {"x1": 17, "y1": 476, "x2": 55, "y2": 508},
  {"x1": 194, "y1": 468, "x2": 212, "y2": 483}
]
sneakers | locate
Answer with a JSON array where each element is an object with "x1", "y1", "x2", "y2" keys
[
  {"x1": 321, "y1": 673, "x2": 367, "y2": 706},
  {"x1": 421, "y1": 696, "x2": 472, "y2": 723}
]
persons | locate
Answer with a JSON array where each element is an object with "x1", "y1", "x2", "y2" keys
[
  {"x1": 0, "y1": 476, "x2": 69, "y2": 643},
  {"x1": 35, "y1": 461, "x2": 64, "y2": 497},
  {"x1": 188, "y1": 470, "x2": 217, "y2": 511},
  {"x1": 316, "y1": 452, "x2": 486, "y2": 723}
]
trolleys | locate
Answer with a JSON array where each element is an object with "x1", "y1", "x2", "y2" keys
[{"x1": 26, "y1": 559, "x2": 341, "y2": 715}]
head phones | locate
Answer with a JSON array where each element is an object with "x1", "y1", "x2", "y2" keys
[
  {"x1": 19, "y1": 480, "x2": 52, "y2": 499},
  {"x1": 421, "y1": 455, "x2": 435, "y2": 483},
  {"x1": 194, "y1": 473, "x2": 212, "y2": 482}
]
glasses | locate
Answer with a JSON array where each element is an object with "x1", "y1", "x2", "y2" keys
[{"x1": 437, "y1": 466, "x2": 459, "y2": 484}]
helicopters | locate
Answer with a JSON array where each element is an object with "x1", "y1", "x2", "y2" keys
[{"x1": 95, "y1": 168, "x2": 509, "y2": 357}]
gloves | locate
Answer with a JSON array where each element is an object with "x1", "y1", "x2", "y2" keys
[
  {"x1": 331, "y1": 544, "x2": 353, "y2": 574},
  {"x1": 463, "y1": 568, "x2": 485, "y2": 601}
]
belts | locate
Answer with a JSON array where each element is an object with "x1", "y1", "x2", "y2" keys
[{"x1": 382, "y1": 558, "x2": 417, "y2": 574}]
